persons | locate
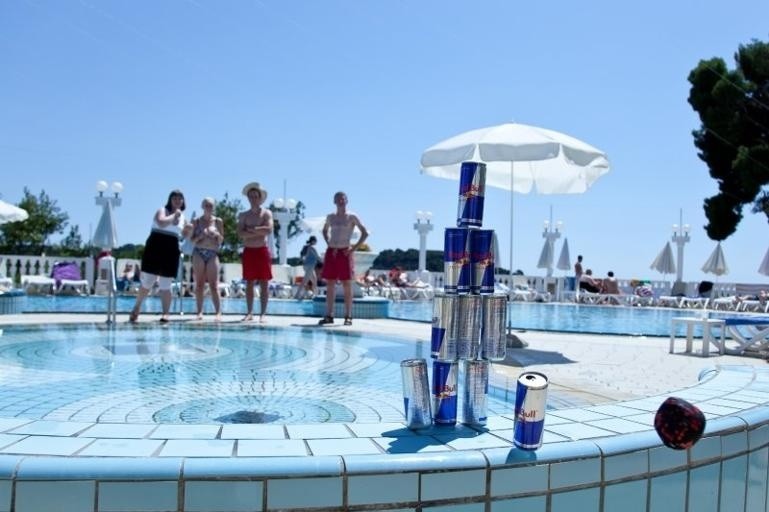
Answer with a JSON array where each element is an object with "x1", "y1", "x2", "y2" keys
[
  {"x1": 130, "y1": 190, "x2": 193, "y2": 323},
  {"x1": 388, "y1": 266, "x2": 429, "y2": 291},
  {"x1": 120, "y1": 260, "x2": 140, "y2": 296},
  {"x1": 575, "y1": 253, "x2": 654, "y2": 309},
  {"x1": 733, "y1": 288, "x2": 769, "y2": 304},
  {"x1": 295, "y1": 235, "x2": 322, "y2": 301},
  {"x1": 236, "y1": 187, "x2": 273, "y2": 323},
  {"x1": 363, "y1": 270, "x2": 388, "y2": 288},
  {"x1": 318, "y1": 192, "x2": 368, "y2": 326},
  {"x1": 187, "y1": 197, "x2": 225, "y2": 324}
]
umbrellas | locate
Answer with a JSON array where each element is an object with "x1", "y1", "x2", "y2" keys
[
  {"x1": 701, "y1": 242, "x2": 729, "y2": 282},
  {"x1": 421, "y1": 120, "x2": 612, "y2": 334},
  {"x1": 756, "y1": 248, "x2": 768, "y2": 277},
  {"x1": 536, "y1": 239, "x2": 547, "y2": 270},
  {"x1": 96, "y1": 200, "x2": 117, "y2": 247},
  {"x1": 556, "y1": 239, "x2": 573, "y2": 271},
  {"x1": 1, "y1": 201, "x2": 28, "y2": 226},
  {"x1": 651, "y1": 241, "x2": 675, "y2": 282}
]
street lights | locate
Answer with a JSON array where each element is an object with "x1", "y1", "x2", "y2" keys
[
  {"x1": 272, "y1": 198, "x2": 298, "y2": 264},
  {"x1": 95, "y1": 180, "x2": 123, "y2": 250},
  {"x1": 671, "y1": 223, "x2": 692, "y2": 284},
  {"x1": 413, "y1": 210, "x2": 434, "y2": 270},
  {"x1": 542, "y1": 219, "x2": 562, "y2": 276}
]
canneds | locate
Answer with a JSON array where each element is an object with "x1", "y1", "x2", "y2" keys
[
  {"x1": 456, "y1": 162, "x2": 487, "y2": 227},
  {"x1": 462, "y1": 360, "x2": 488, "y2": 424},
  {"x1": 458, "y1": 296, "x2": 481, "y2": 360},
  {"x1": 431, "y1": 293, "x2": 458, "y2": 363},
  {"x1": 443, "y1": 227, "x2": 471, "y2": 293},
  {"x1": 400, "y1": 358, "x2": 433, "y2": 429},
  {"x1": 470, "y1": 230, "x2": 496, "y2": 296},
  {"x1": 433, "y1": 360, "x2": 458, "y2": 425},
  {"x1": 512, "y1": 371, "x2": 548, "y2": 450},
  {"x1": 481, "y1": 293, "x2": 506, "y2": 361}
]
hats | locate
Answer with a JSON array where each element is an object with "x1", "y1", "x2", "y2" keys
[{"x1": 241, "y1": 181, "x2": 269, "y2": 204}]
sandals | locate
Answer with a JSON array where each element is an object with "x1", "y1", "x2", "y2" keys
[
  {"x1": 318, "y1": 315, "x2": 334, "y2": 325},
  {"x1": 343, "y1": 316, "x2": 354, "y2": 326}
]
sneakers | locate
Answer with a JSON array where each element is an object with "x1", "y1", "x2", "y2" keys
[
  {"x1": 159, "y1": 318, "x2": 170, "y2": 323},
  {"x1": 129, "y1": 310, "x2": 139, "y2": 321}
]
